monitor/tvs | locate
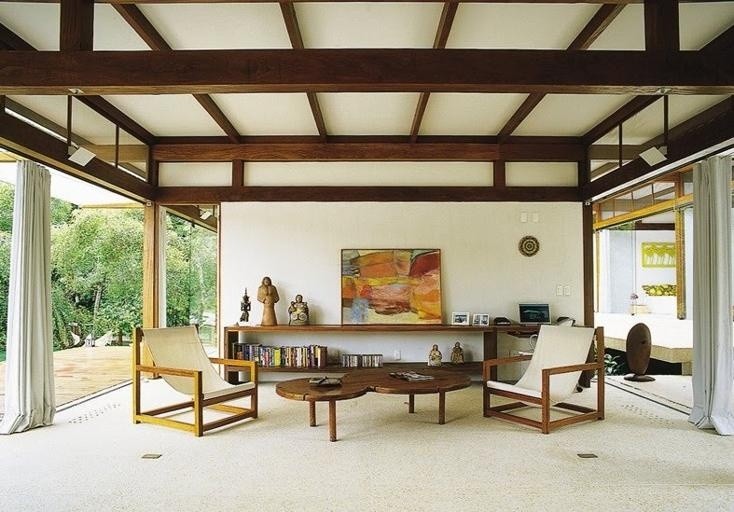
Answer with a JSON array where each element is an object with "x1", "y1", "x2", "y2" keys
[{"x1": 518, "y1": 303, "x2": 551, "y2": 326}]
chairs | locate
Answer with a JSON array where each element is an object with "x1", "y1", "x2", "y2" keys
[
  {"x1": 482, "y1": 326, "x2": 605, "y2": 433},
  {"x1": 132, "y1": 324, "x2": 258, "y2": 438}
]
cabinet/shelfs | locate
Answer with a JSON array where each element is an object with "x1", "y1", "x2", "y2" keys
[{"x1": 224, "y1": 325, "x2": 541, "y2": 384}]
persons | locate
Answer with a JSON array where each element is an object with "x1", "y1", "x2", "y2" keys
[
  {"x1": 427, "y1": 344, "x2": 442, "y2": 367},
  {"x1": 450, "y1": 341, "x2": 464, "y2": 364},
  {"x1": 239, "y1": 296, "x2": 251, "y2": 322},
  {"x1": 287, "y1": 294, "x2": 308, "y2": 325},
  {"x1": 256, "y1": 276, "x2": 280, "y2": 325}
]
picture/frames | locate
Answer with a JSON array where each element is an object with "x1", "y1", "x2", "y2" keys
[
  {"x1": 341, "y1": 248, "x2": 443, "y2": 325},
  {"x1": 473, "y1": 314, "x2": 489, "y2": 326},
  {"x1": 451, "y1": 312, "x2": 469, "y2": 326},
  {"x1": 642, "y1": 242, "x2": 675, "y2": 268}
]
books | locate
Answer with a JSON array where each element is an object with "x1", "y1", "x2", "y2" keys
[
  {"x1": 494, "y1": 317, "x2": 510, "y2": 325},
  {"x1": 309, "y1": 377, "x2": 342, "y2": 387},
  {"x1": 231, "y1": 341, "x2": 327, "y2": 368},
  {"x1": 341, "y1": 354, "x2": 383, "y2": 368},
  {"x1": 389, "y1": 368, "x2": 434, "y2": 382}
]
